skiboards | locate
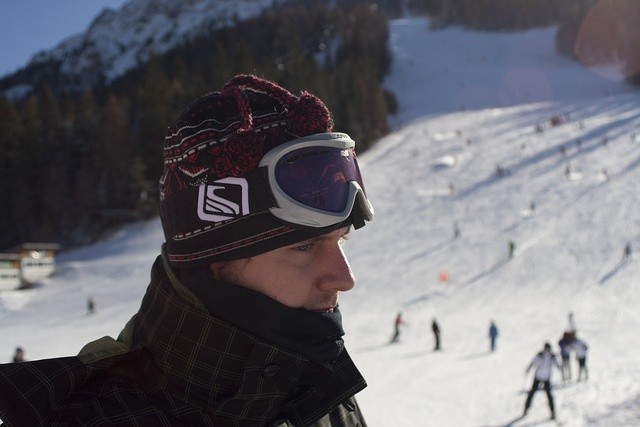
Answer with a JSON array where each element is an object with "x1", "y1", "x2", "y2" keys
[{"x1": 506, "y1": 416, "x2": 562, "y2": 427}]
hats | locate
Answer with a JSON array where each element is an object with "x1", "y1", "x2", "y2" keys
[{"x1": 158, "y1": 73, "x2": 366, "y2": 267}]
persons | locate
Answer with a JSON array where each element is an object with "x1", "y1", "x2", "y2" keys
[
  {"x1": 569, "y1": 337, "x2": 589, "y2": 382},
  {"x1": 560, "y1": 332, "x2": 571, "y2": 381},
  {"x1": 489, "y1": 323, "x2": 497, "y2": 351},
  {"x1": 14, "y1": 349, "x2": 23, "y2": 363},
  {"x1": 433, "y1": 320, "x2": 440, "y2": 350},
  {"x1": 569, "y1": 313, "x2": 578, "y2": 337},
  {"x1": 393, "y1": 313, "x2": 403, "y2": 340},
  {"x1": 523, "y1": 343, "x2": 562, "y2": 419},
  {"x1": 0, "y1": 74, "x2": 373, "y2": 427}
]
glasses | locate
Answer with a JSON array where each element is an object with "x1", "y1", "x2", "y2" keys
[{"x1": 159, "y1": 132, "x2": 375, "y2": 242}]
